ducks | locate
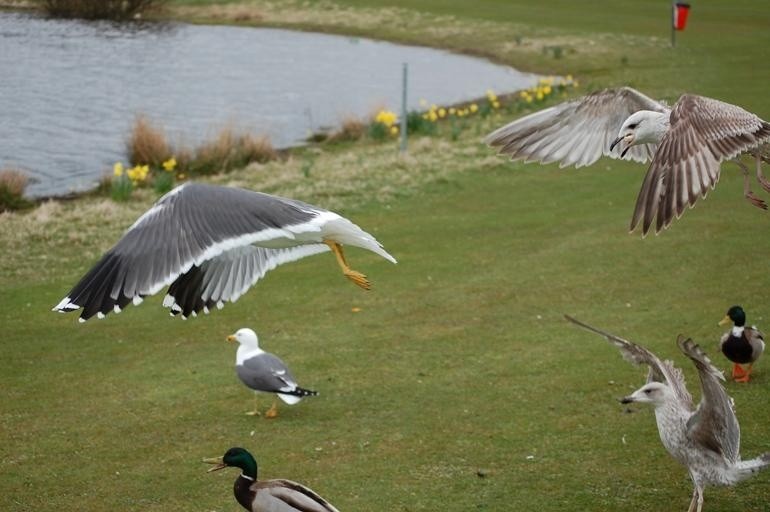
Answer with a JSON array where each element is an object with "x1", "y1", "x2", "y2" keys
[
  {"x1": 715, "y1": 300, "x2": 766, "y2": 385},
  {"x1": 199, "y1": 444, "x2": 340, "y2": 511}
]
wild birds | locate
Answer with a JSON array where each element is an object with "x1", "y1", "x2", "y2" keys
[
  {"x1": 482, "y1": 85, "x2": 770, "y2": 240},
  {"x1": 49, "y1": 179, "x2": 400, "y2": 324},
  {"x1": 224, "y1": 328, "x2": 319, "y2": 420},
  {"x1": 559, "y1": 310, "x2": 769, "y2": 512}
]
